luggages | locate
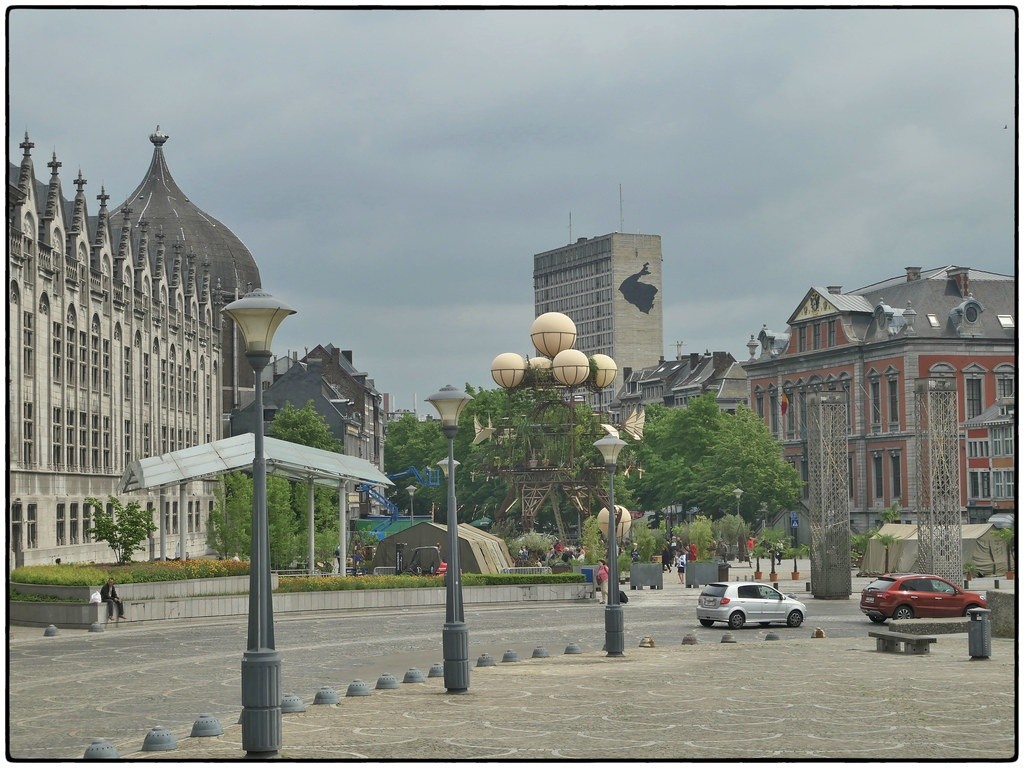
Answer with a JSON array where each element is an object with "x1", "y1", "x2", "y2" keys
[{"x1": 620, "y1": 591, "x2": 628, "y2": 604}]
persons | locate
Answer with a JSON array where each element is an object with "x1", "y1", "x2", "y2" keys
[
  {"x1": 100, "y1": 578, "x2": 126, "y2": 620},
  {"x1": 597, "y1": 559, "x2": 609, "y2": 604},
  {"x1": 748, "y1": 537, "x2": 756, "y2": 561},
  {"x1": 662, "y1": 548, "x2": 671, "y2": 573},
  {"x1": 233, "y1": 553, "x2": 240, "y2": 561},
  {"x1": 631, "y1": 542, "x2": 641, "y2": 563},
  {"x1": 676, "y1": 550, "x2": 685, "y2": 584},
  {"x1": 521, "y1": 540, "x2": 585, "y2": 567}
]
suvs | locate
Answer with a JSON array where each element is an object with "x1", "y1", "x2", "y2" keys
[{"x1": 858, "y1": 573, "x2": 989, "y2": 623}]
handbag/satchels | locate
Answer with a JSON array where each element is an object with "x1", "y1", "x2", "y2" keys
[{"x1": 596, "y1": 575, "x2": 602, "y2": 586}]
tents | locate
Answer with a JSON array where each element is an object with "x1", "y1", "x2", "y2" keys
[
  {"x1": 860, "y1": 522, "x2": 1014, "y2": 578},
  {"x1": 367, "y1": 521, "x2": 514, "y2": 574}
]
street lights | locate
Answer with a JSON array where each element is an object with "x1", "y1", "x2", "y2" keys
[
  {"x1": 733, "y1": 488, "x2": 744, "y2": 517},
  {"x1": 405, "y1": 484, "x2": 414, "y2": 527},
  {"x1": 423, "y1": 383, "x2": 479, "y2": 692},
  {"x1": 590, "y1": 433, "x2": 637, "y2": 657},
  {"x1": 222, "y1": 285, "x2": 297, "y2": 758}
]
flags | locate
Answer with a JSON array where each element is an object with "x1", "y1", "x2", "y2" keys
[{"x1": 782, "y1": 388, "x2": 788, "y2": 415}]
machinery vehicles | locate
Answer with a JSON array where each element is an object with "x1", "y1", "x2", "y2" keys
[{"x1": 358, "y1": 465, "x2": 440, "y2": 543}]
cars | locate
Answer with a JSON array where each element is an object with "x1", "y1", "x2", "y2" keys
[{"x1": 695, "y1": 582, "x2": 806, "y2": 630}]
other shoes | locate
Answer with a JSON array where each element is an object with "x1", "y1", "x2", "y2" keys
[
  {"x1": 108, "y1": 616, "x2": 114, "y2": 620},
  {"x1": 600, "y1": 601, "x2": 606, "y2": 604},
  {"x1": 118, "y1": 615, "x2": 127, "y2": 620}
]
